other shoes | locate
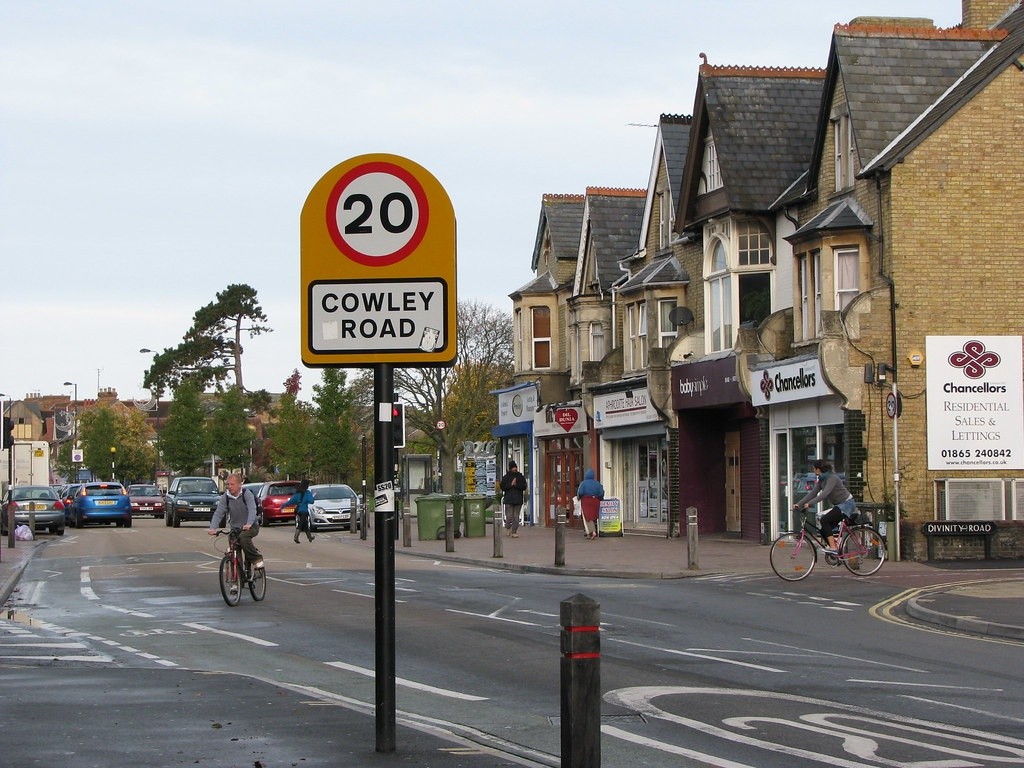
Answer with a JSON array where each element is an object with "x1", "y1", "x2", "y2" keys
[
  {"x1": 310, "y1": 535, "x2": 315, "y2": 542},
  {"x1": 294, "y1": 537, "x2": 300, "y2": 544},
  {"x1": 590, "y1": 534, "x2": 596, "y2": 540},
  {"x1": 230, "y1": 585, "x2": 237, "y2": 592},
  {"x1": 512, "y1": 533, "x2": 519, "y2": 538},
  {"x1": 822, "y1": 546, "x2": 839, "y2": 555},
  {"x1": 507, "y1": 527, "x2": 511, "y2": 537},
  {"x1": 256, "y1": 561, "x2": 265, "y2": 568}
]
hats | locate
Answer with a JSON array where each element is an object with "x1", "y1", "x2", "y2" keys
[{"x1": 509, "y1": 460, "x2": 517, "y2": 469}]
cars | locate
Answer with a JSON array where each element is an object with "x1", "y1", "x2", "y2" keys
[
  {"x1": 48, "y1": 483, "x2": 80, "y2": 526},
  {"x1": 126, "y1": 484, "x2": 165, "y2": 519},
  {"x1": 256, "y1": 481, "x2": 302, "y2": 527},
  {"x1": 295, "y1": 484, "x2": 363, "y2": 532},
  {"x1": 67, "y1": 482, "x2": 132, "y2": 529},
  {"x1": 0, "y1": 485, "x2": 66, "y2": 536},
  {"x1": 241, "y1": 482, "x2": 264, "y2": 499}
]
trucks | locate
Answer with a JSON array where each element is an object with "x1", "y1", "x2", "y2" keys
[{"x1": 0, "y1": 440, "x2": 50, "y2": 499}]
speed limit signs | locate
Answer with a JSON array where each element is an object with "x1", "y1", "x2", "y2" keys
[
  {"x1": 886, "y1": 393, "x2": 896, "y2": 418},
  {"x1": 436, "y1": 420, "x2": 446, "y2": 430},
  {"x1": 326, "y1": 162, "x2": 428, "y2": 266}
]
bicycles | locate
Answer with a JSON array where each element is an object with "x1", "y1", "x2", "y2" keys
[
  {"x1": 769, "y1": 504, "x2": 888, "y2": 582},
  {"x1": 207, "y1": 525, "x2": 267, "y2": 607}
]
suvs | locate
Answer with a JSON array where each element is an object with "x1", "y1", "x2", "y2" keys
[{"x1": 164, "y1": 477, "x2": 226, "y2": 529}]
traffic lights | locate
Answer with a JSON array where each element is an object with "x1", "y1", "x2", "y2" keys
[
  {"x1": 3, "y1": 417, "x2": 14, "y2": 450},
  {"x1": 393, "y1": 402, "x2": 405, "y2": 448}
]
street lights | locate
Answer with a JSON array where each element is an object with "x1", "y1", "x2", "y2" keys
[
  {"x1": 63, "y1": 382, "x2": 77, "y2": 484},
  {"x1": 0, "y1": 393, "x2": 11, "y2": 418},
  {"x1": 140, "y1": 348, "x2": 161, "y2": 472},
  {"x1": 111, "y1": 447, "x2": 116, "y2": 482}
]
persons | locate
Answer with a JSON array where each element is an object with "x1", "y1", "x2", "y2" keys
[
  {"x1": 242, "y1": 475, "x2": 252, "y2": 484},
  {"x1": 281, "y1": 479, "x2": 316, "y2": 544},
  {"x1": 208, "y1": 472, "x2": 264, "y2": 595},
  {"x1": 500, "y1": 461, "x2": 527, "y2": 537},
  {"x1": 577, "y1": 469, "x2": 605, "y2": 540},
  {"x1": 792, "y1": 458, "x2": 856, "y2": 568}
]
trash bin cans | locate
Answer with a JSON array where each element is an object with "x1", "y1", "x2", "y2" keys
[
  {"x1": 369, "y1": 496, "x2": 374, "y2": 512},
  {"x1": 414, "y1": 496, "x2": 447, "y2": 541},
  {"x1": 440, "y1": 494, "x2": 467, "y2": 539},
  {"x1": 463, "y1": 496, "x2": 486, "y2": 538}
]
